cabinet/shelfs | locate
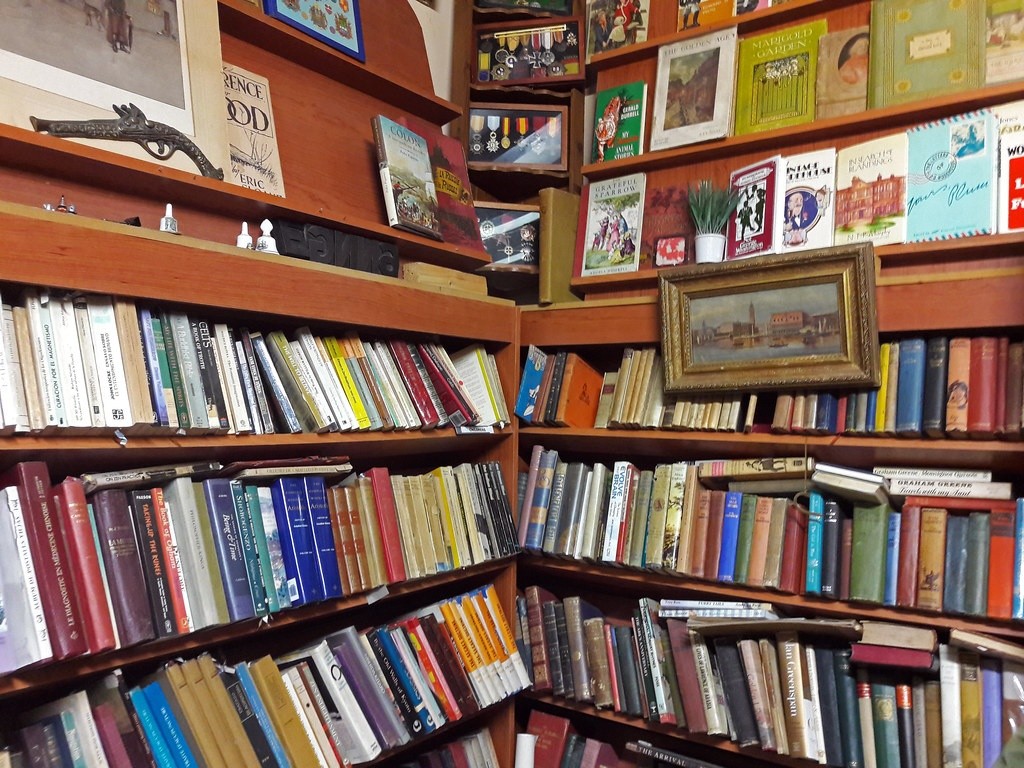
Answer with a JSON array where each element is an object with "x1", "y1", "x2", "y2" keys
[
  {"x1": 509, "y1": 0, "x2": 1024, "y2": 768},
  {"x1": 0, "y1": 0, "x2": 518, "y2": 768},
  {"x1": 468, "y1": 84, "x2": 585, "y2": 303}
]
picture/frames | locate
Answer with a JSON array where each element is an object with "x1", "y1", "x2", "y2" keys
[
  {"x1": 656, "y1": 241, "x2": 883, "y2": 394},
  {"x1": 465, "y1": 201, "x2": 544, "y2": 280},
  {"x1": 470, "y1": 0, "x2": 592, "y2": 82},
  {"x1": 469, "y1": 101, "x2": 568, "y2": 172},
  {"x1": 466, "y1": 17, "x2": 585, "y2": 81}
]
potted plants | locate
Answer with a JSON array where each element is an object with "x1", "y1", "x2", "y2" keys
[{"x1": 685, "y1": 176, "x2": 747, "y2": 265}]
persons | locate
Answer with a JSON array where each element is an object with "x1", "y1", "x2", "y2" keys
[
  {"x1": 604, "y1": 14, "x2": 627, "y2": 52},
  {"x1": 594, "y1": 8, "x2": 608, "y2": 52},
  {"x1": 614, "y1": 1, "x2": 635, "y2": 31},
  {"x1": 683, "y1": 0, "x2": 701, "y2": 29},
  {"x1": 786, "y1": 194, "x2": 814, "y2": 229},
  {"x1": 628, "y1": 1, "x2": 643, "y2": 29}
]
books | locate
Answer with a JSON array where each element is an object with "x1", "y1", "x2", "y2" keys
[{"x1": 0, "y1": 0, "x2": 1024, "y2": 768}]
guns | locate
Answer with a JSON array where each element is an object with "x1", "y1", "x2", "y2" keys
[{"x1": 30, "y1": 101, "x2": 224, "y2": 181}]
